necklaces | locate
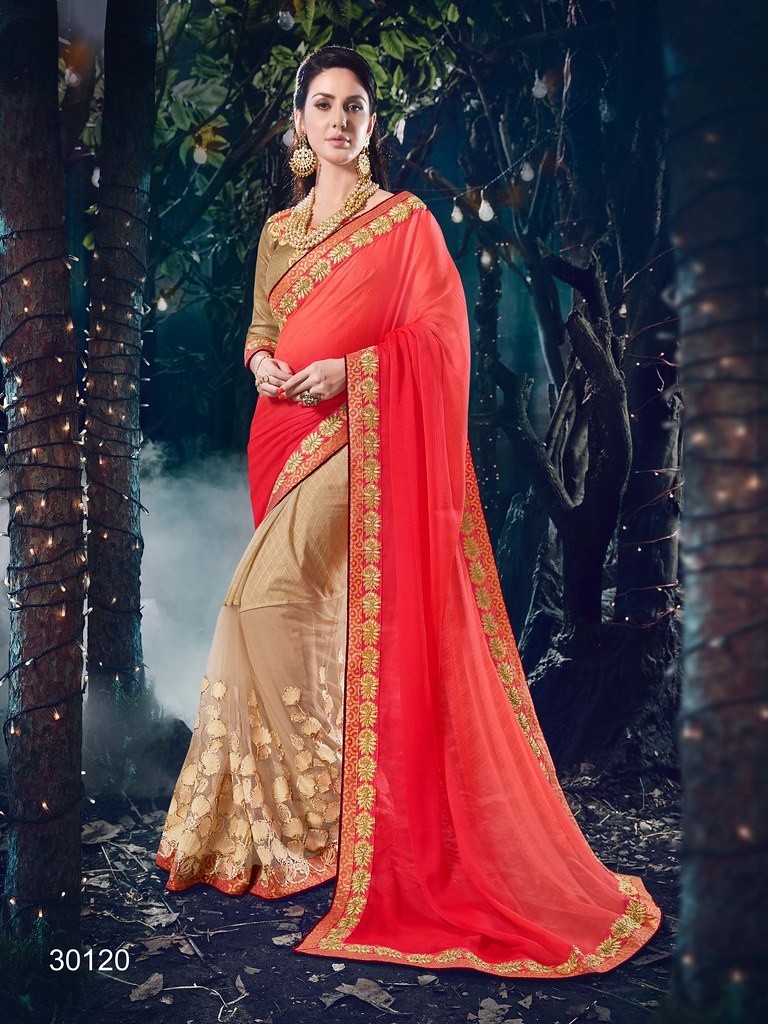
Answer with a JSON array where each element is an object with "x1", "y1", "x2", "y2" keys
[{"x1": 285, "y1": 178, "x2": 379, "y2": 250}]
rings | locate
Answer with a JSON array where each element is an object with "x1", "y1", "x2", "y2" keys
[
  {"x1": 254, "y1": 355, "x2": 272, "y2": 374},
  {"x1": 300, "y1": 390, "x2": 320, "y2": 405},
  {"x1": 252, "y1": 375, "x2": 270, "y2": 386}
]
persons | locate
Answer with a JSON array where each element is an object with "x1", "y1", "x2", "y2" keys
[{"x1": 155, "y1": 42, "x2": 662, "y2": 979}]
